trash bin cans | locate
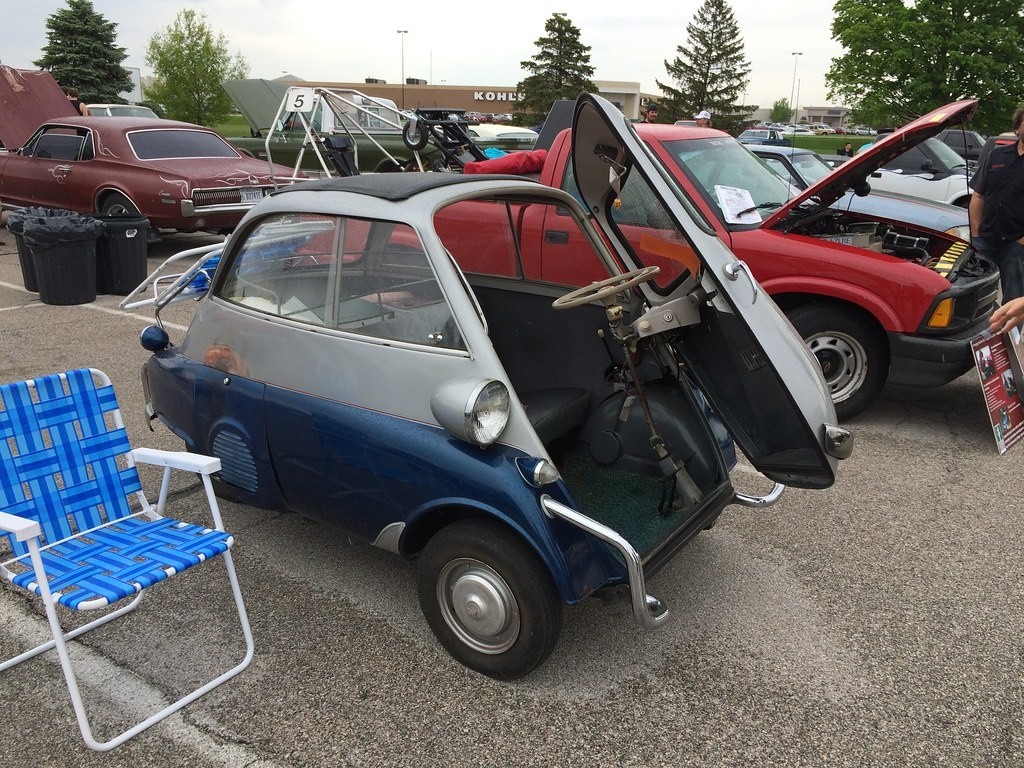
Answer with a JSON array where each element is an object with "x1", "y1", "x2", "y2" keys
[
  {"x1": 92, "y1": 213, "x2": 150, "y2": 294},
  {"x1": 22, "y1": 217, "x2": 102, "y2": 305},
  {"x1": 5, "y1": 206, "x2": 79, "y2": 292}
]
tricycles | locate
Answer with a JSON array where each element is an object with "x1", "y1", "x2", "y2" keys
[{"x1": 142, "y1": 92, "x2": 855, "y2": 682}]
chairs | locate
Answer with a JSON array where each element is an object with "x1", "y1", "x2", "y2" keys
[{"x1": 0, "y1": 367, "x2": 255, "y2": 753}]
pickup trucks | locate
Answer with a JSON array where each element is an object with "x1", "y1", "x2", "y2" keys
[{"x1": 288, "y1": 99, "x2": 1001, "y2": 421}]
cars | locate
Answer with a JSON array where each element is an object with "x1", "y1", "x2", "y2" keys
[
  {"x1": 736, "y1": 120, "x2": 877, "y2": 145},
  {"x1": 935, "y1": 130, "x2": 1004, "y2": 161},
  {"x1": 0, "y1": 68, "x2": 310, "y2": 240},
  {"x1": 85, "y1": 103, "x2": 159, "y2": 119},
  {"x1": 220, "y1": 76, "x2": 541, "y2": 172},
  {"x1": 862, "y1": 131, "x2": 977, "y2": 208},
  {"x1": 466, "y1": 111, "x2": 516, "y2": 125}
]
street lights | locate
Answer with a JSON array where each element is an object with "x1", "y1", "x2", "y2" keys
[
  {"x1": 788, "y1": 53, "x2": 802, "y2": 124},
  {"x1": 397, "y1": 31, "x2": 408, "y2": 111}
]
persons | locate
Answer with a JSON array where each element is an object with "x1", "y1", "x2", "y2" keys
[
  {"x1": 639, "y1": 103, "x2": 657, "y2": 124},
  {"x1": 1001, "y1": 409, "x2": 1010, "y2": 431},
  {"x1": 1004, "y1": 374, "x2": 1015, "y2": 391},
  {"x1": 856, "y1": 133, "x2": 889, "y2": 153},
  {"x1": 65, "y1": 88, "x2": 88, "y2": 116},
  {"x1": 837, "y1": 142, "x2": 853, "y2": 157},
  {"x1": 983, "y1": 356, "x2": 995, "y2": 378},
  {"x1": 967, "y1": 103, "x2": 1024, "y2": 334},
  {"x1": 995, "y1": 426, "x2": 1002, "y2": 442},
  {"x1": 694, "y1": 112, "x2": 711, "y2": 129}
]
